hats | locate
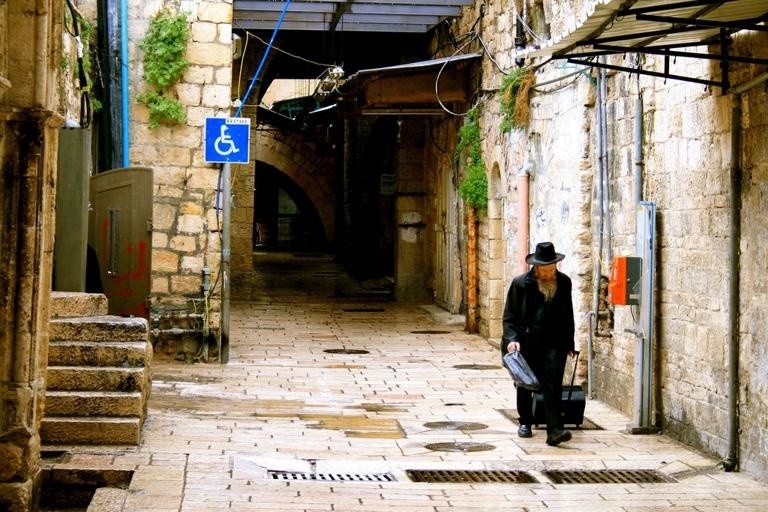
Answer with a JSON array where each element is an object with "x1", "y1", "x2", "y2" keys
[{"x1": 526, "y1": 242, "x2": 565, "y2": 265}]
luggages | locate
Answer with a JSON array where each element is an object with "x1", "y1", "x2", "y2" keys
[{"x1": 532, "y1": 351, "x2": 585, "y2": 427}]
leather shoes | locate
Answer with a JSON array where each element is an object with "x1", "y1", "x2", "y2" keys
[
  {"x1": 518, "y1": 425, "x2": 532, "y2": 437},
  {"x1": 547, "y1": 431, "x2": 571, "y2": 445}
]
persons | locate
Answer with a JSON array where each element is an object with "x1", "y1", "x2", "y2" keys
[{"x1": 501, "y1": 242, "x2": 575, "y2": 445}]
